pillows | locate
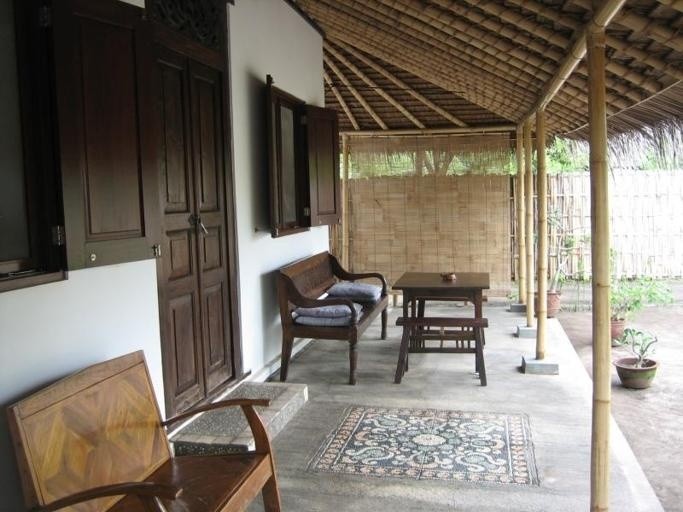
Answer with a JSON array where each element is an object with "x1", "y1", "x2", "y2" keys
[
  {"x1": 296, "y1": 315, "x2": 364, "y2": 325},
  {"x1": 293, "y1": 303, "x2": 364, "y2": 316},
  {"x1": 329, "y1": 283, "x2": 382, "y2": 303}
]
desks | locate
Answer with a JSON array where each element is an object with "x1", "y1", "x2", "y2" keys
[{"x1": 393, "y1": 271, "x2": 490, "y2": 350}]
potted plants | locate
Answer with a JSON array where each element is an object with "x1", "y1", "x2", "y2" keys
[
  {"x1": 533, "y1": 207, "x2": 590, "y2": 318},
  {"x1": 609, "y1": 273, "x2": 674, "y2": 344},
  {"x1": 611, "y1": 327, "x2": 661, "y2": 390}
]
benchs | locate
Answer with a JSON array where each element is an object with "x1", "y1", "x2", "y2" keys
[
  {"x1": 276, "y1": 251, "x2": 389, "y2": 386},
  {"x1": 6, "y1": 349, "x2": 285, "y2": 512},
  {"x1": 393, "y1": 317, "x2": 489, "y2": 386}
]
